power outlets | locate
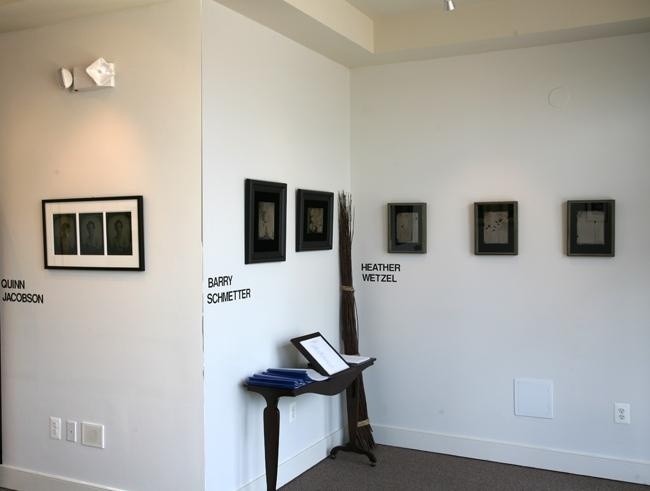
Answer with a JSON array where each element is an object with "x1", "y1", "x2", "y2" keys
[
  {"x1": 50, "y1": 417, "x2": 62, "y2": 438},
  {"x1": 615, "y1": 403, "x2": 630, "y2": 424}
]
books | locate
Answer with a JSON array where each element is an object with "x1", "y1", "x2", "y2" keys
[{"x1": 245, "y1": 332, "x2": 370, "y2": 391}]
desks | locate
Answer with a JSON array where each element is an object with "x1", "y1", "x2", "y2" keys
[{"x1": 244, "y1": 353, "x2": 377, "y2": 491}]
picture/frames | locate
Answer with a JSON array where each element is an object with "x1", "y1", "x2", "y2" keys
[
  {"x1": 566, "y1": 198, "x2": 615, "y2": 257},
  {"x1": 472, "y1": 200, "x2": 519, "y2": 255},
  {"x1": 41, "y1": 194, "x2": 146, "y2": 270},
  {"x1": 296, "y1": 189, "x2": 334, "y2": 252},
  {"x1": 388, "y1": 201, "x2": 428, "y2": 255},
  {"x1": 246, "y1": 179, "x2": 286, "y2": 264}
]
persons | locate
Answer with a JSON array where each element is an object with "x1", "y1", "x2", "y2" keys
[{"x1": 57, "y1": 218, "x2": 132, "y2": 256}]
